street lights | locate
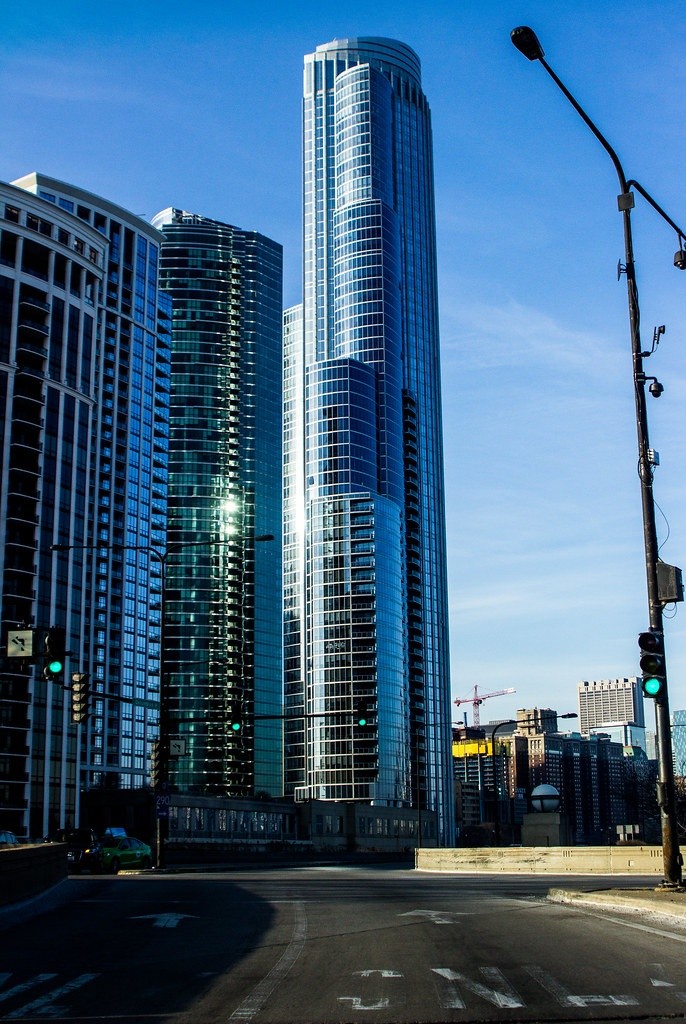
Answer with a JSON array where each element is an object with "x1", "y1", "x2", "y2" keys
[
  {"x1": 509, "y1": 25, "x2": 684, "y2": 886},
  {"x1": 492, "y1": 713, "x2": 578, "y2": 846}
]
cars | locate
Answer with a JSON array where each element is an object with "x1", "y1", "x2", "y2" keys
[
  {"x1": 42, "y1": 829, "x2": 104, "y2": 875},
  {"x1": 98, "y1": 835, "x2": 153, "y2": 874}
]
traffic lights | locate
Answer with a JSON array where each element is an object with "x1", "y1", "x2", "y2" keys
[
  {"x1": 42, "y1": 628, "x2": 63, "y2": 681},
  {"x1": 231, "y1": 703, "x2": 241, "y2": 731},
  {"x1": 357, "y1": 701, "x2": 367, "y2": 729},
  {"x1": 639, "y1": 632, "x2": 666, "y2": 699}
]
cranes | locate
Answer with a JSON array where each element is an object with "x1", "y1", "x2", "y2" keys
[{"x1": 453, "y1": 684, "x2": 517, "y2": 726}]
